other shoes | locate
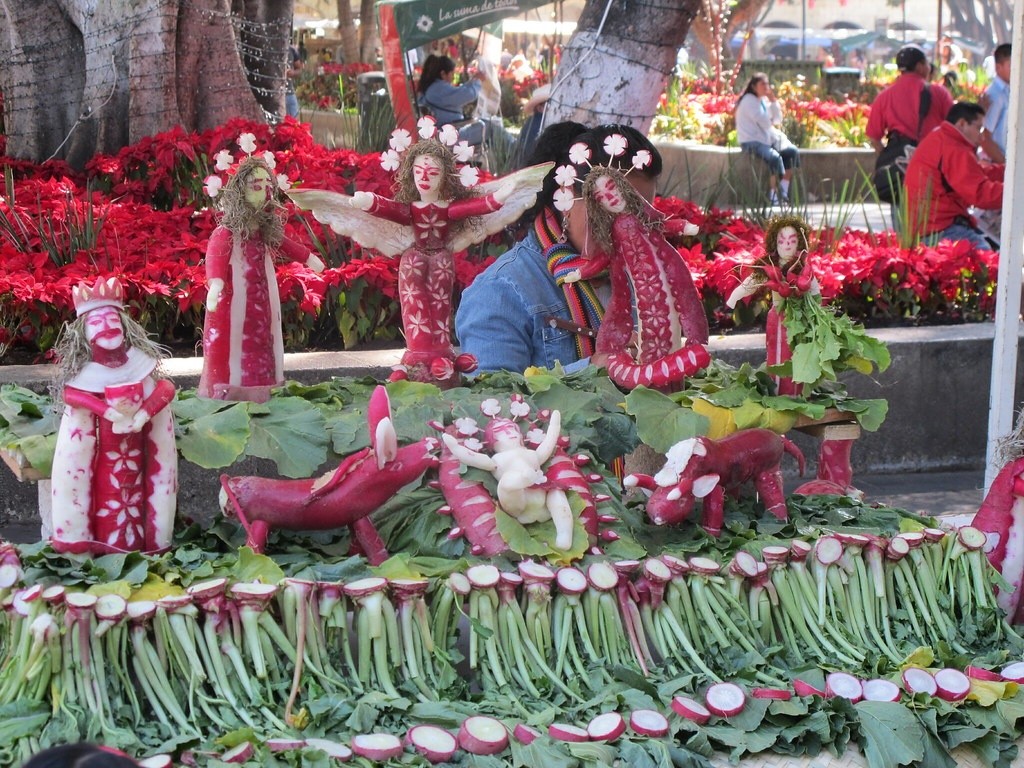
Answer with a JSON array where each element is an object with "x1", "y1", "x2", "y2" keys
[
  {"x1": 769, "y1": 196, "x2": 787, "y2": 206},
  {"x1": 784, "y1": 196, "x2": 796, "y2": 206}
]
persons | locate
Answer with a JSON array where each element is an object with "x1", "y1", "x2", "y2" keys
[
  {"x1": 727, "y1": 217, "x2": 822, "y2": 399},
  {"x1": 735, "y1": 73, "x2": 800, "y2": 206},
  {"x1": 975, "y1": 43, "x2": 1013, "y2": 161},
  {"x1": 349, "y1": 139, "x2": 527, "y2": 377},
  {"x1": 317, "y1": 48, "x2": 333, "y2": 64},
  {"x1": 417, "y1": 54, "x2": 517, "y2": 147},
  {"x1": 900, "y1": 102, "x2": 1004, "y2": 252},
  {"x1": 816, "y1": 45, "x2": 864, "y2": 68},
  {"x1": 51, "y1": 282, "x2": 179, "y2": 547},
  {"x1": 558, "y1": 166, "x2": 708, "y2": 388},
  {"x1": 285, "y1": 41, "x2": 307, "y2": 121},
  {"x1": 195, "y1": 159, "x2": 325, "y2": 391},
  {"x1": 455, "y1": 121, "x2": 662, "y2": 377},
  {"x1": 510, "y1": 85, "x2": 551, "y2": 168},
  {"x1": 442, "y1": 409, "x2": 574, "y2": 551},
  {"x1": 867, "y1": 44, "x2": 952, "y2": 234},
  {"x1": 927, "y1": 33, "x2": 963, "y2": 87}
]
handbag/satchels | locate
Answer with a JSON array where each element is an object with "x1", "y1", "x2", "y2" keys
[{"x1": 874, "y1": 130, "x2": 919, "y2": 207}]
